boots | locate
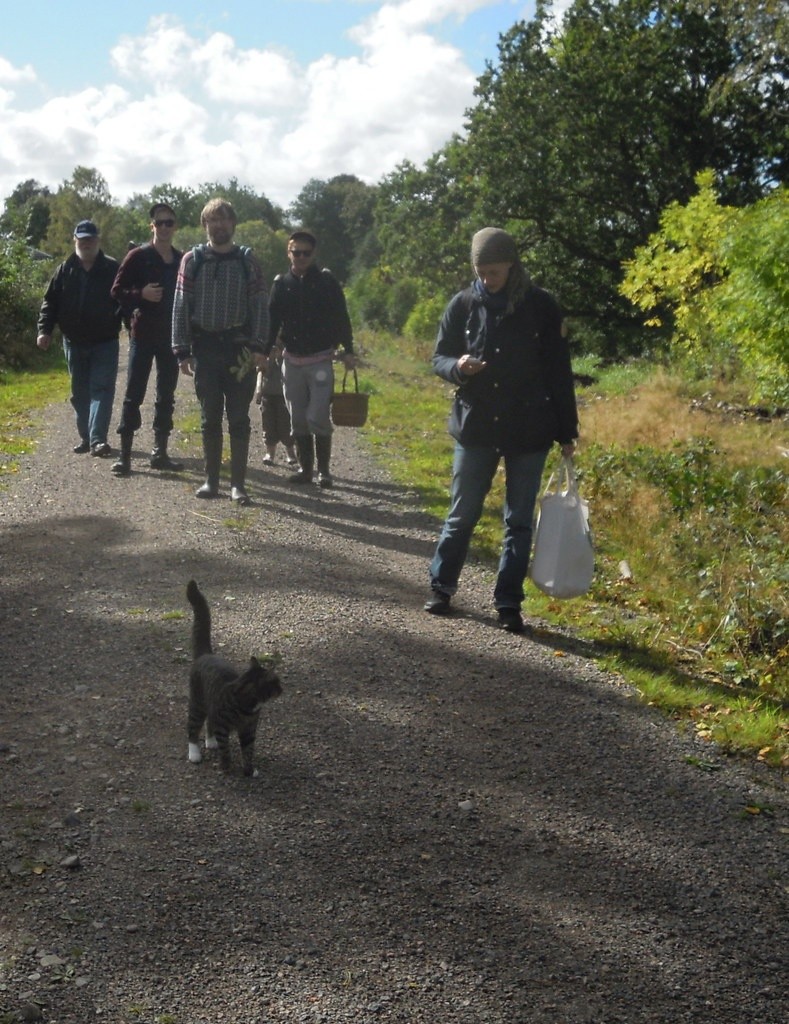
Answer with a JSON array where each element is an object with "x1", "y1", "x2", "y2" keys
[
  {"x1": 289, "y1": 434, "x2": 314, "y2": 484},
  {"x1": 194, "y1": 434, "x2": 223, "y2": 498},
  {"x1": 314, "y1": 434, "x2": 333, "y2": 487},
  {"x1": 284, "y1": 444, "x2": 297, "y2": 463},
  {"x1": 229, "y1": 434, "x2": 249, "y2": 504},
  {"x1": 151, "y1": 433, "x2": 186, "y2": 472},
  {"x1": 109, "y1": 437, "x2": 131, "y2": 472},
  {"x1": 262, "y1": 445, "x2": 276, "y2": 464}
]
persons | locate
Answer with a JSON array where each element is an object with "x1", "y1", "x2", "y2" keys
[
  {"x1": 423, "y1": 225, "x2": 580, "y2": 630},
  {"x1": 263, "y1": 232, "x2": 354, "y2": 488},
  {"x1": 254, "y1": 356, "x2": 297, "y2": 466},
  {"x1": 36, "y1": 218, "x2": 132, "y2": 455},
  {"x1": 169, "y1": 197, "x2": 269, "y2": 505},
  {"x1": 110, "y1": 203, "x2": 185, "y2": 473}
]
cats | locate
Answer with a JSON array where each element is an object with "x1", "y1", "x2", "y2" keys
[{"x1": 185, "y1": 577, "x2": 283, "y2": 779}]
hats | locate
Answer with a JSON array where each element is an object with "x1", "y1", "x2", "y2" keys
[
  {"x1": 150, "y1": 204, "x2": 177, "y2": 218},
  {"x1": 472, "y1": 227, "x2": 514, "y2": 266},
  {"x1": 290, "y1": 231, "x2": 316, "y2": 248},
  {"x1": 74, "y1": 219, "x2": 98, "y2": 238}
]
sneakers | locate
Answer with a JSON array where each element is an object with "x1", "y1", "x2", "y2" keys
[
  {"x1": 73, "y1": 440, "x2": 90, "y2": 453},
  {"x1": 423, "y1": 590, "x2": 452, "y2": 615},
  {"x1": 91, "y1": 443, "x2": 111, "y2": 456},
  {"x1": 498, "y1": 607, "x2": 524, "y2": 632}
]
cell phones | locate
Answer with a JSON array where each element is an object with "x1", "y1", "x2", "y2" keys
[{"x1": 465, "y1": 356, "x2": 481, "y2": 366}]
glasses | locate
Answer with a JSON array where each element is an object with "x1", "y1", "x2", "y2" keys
[
  {"x1": 290, "y1": 249, "x2": 313, "y2": 257},
  {"x1": 155, "y1": 220, "x2": 175, "y2": 227}
]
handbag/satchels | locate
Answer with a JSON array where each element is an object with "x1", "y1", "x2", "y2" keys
[
  {"x1": 524, "y1": 453, "x2": 593, "y2": 599},
  {"x1": 332, "y1": 367, "x2": 370, "y2": 427}
]
